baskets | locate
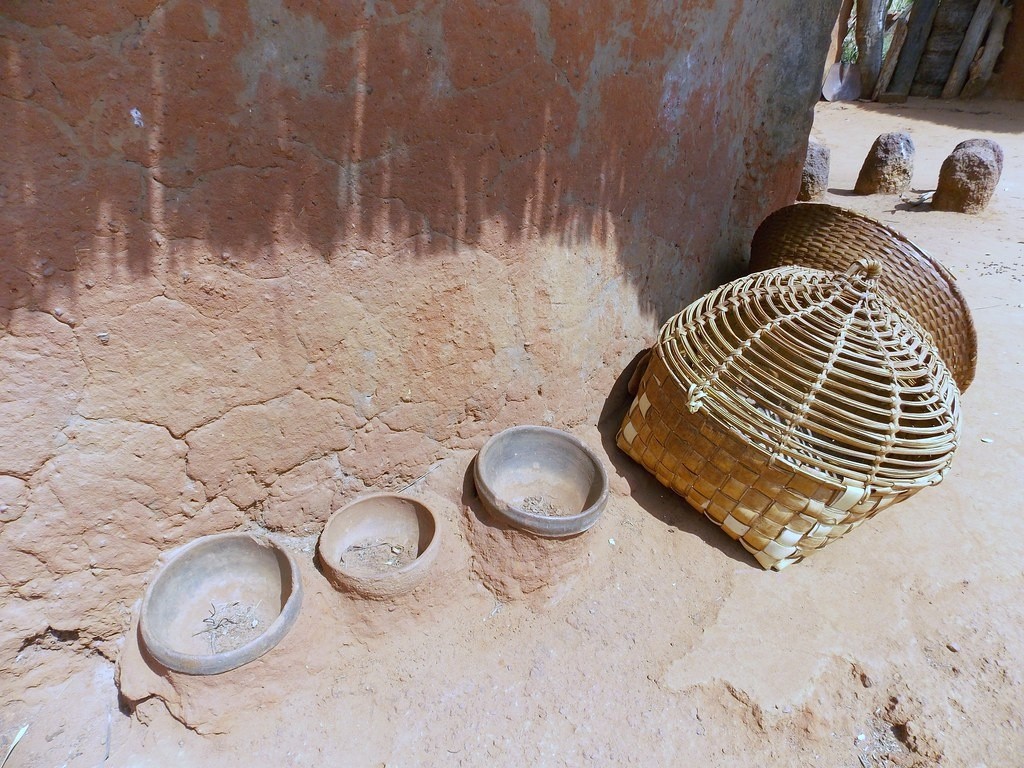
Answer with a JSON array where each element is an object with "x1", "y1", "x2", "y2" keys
[
  {"x1": 617, "y1": 258, "x2": 960, "y2": 570},
  {"x1": 753, "y1": 203, "x2": 977, "y2": 394}
]
